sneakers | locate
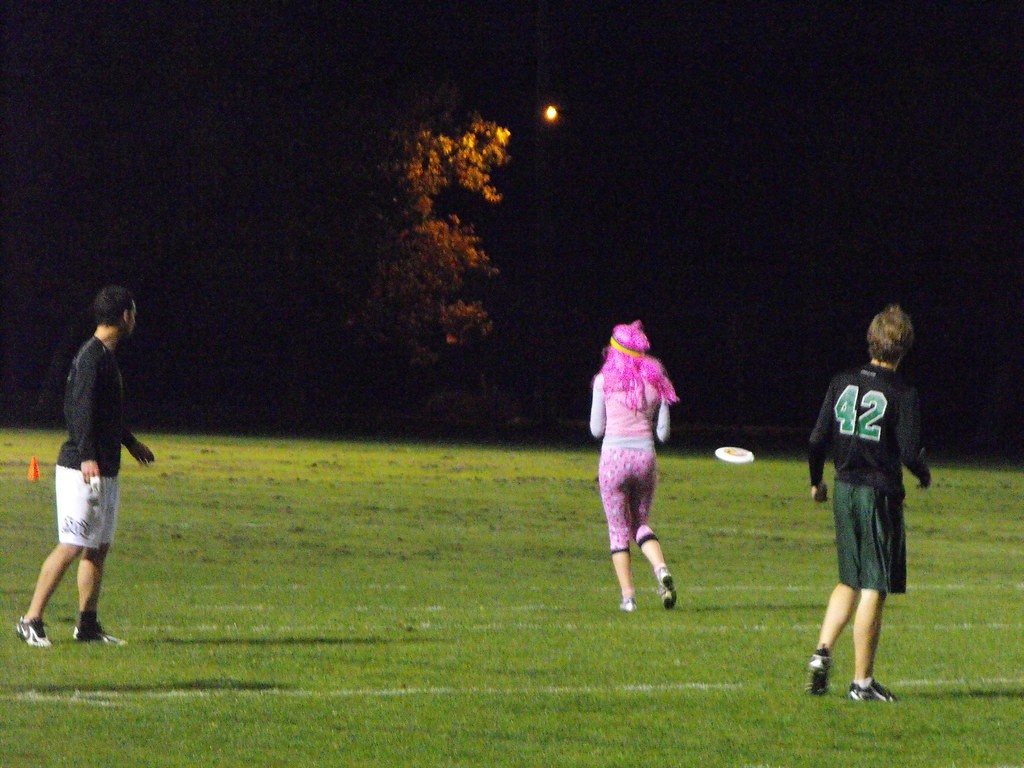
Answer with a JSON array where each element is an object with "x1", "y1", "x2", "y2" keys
[
  {"x1": 73, "y1": 624, "x2": 125, "y2": 645},
  {"x1": 848, "y1": 678, "x2": 897, "y2": 703},
  {"x1": 656, "y1": 568, "x2": 676, "y2": 610},
  {"x1": 620, "y1": 597, "x2": 637, "y2": 612},
  {"x1": 17, "y1": 616, "x2": 53, "y2": 647},
  {"x1": 805, "y1": 648, "x2": 832, "y2": 695}
]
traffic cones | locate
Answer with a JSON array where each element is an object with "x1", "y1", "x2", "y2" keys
[{"x1": 26, "y1": 456, "x2": 41, "y2": 482}]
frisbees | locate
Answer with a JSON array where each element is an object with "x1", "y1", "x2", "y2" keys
[{"x1": 715, "y1": 446, "x2": 755, "y2": 464}]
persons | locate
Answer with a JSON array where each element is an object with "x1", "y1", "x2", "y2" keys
[
  {"x1": 15, "y1": 283, "x2": 154, "y2": 647},
  {"x1": 804, "y1": 305, "x2": 932, "y2": 702},
  {"x1": 590, "y1": 321, "x2": 680, "y2": 611}
]
